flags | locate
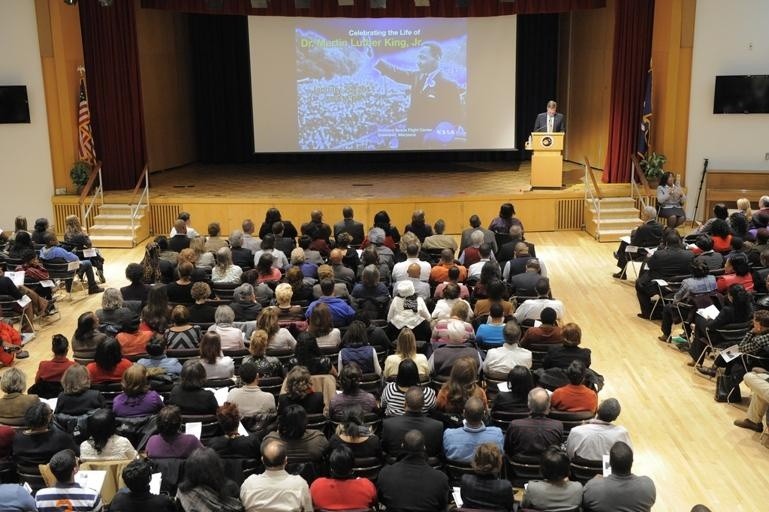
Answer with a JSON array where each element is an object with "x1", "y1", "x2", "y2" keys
[
  {"x1": 78, "y1": 77, "x2": 97, "y2": 166},
  {"x1": 637, "y1": 69, "x2": 653, "y2": 161}
]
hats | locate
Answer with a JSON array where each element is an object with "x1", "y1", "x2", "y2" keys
[{"x1": 397, "y1": 279, "x2": 415, "y2": 297}]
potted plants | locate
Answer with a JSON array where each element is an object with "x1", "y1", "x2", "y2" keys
[
  {"x1": 70, "y1": 159, "x2": 92, "y2": 195},
  {"x1": 637, "y1": 152, "x2": 667, "y2": 189}
]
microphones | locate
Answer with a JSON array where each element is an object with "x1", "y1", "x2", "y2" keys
[{"x1": 537, "y1": 120, "x2": 550, "y2": 131}]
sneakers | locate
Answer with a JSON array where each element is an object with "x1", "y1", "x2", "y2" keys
[
  {"x1": 97, "y1": 269, "x2": 105, "y2": 283},
  {"x1": 698, "y1": 365, "x2": 715, "y2": 378},
  {"x1": 613, "y1": 272, "x2": 627, "y2": 279},
  {"x1": 638, "y1": 313, "x2": 653, "y2": 319},
  {"x1": 19, "y1": 332, "x2": 37, "y2": 346},
  {"x1": 734, "y1": 419, "x2": 764, "y2": 433},
  {"x1": 659, "y1": 335, "x2": 671, "y2": 342},
  {"x1": 687, "y1": 361, "x2": 701, "y2": 366},
  {"x1": 45, "y1": 297, "x2": 57, "y2": 314},
  {"x1": 89, "y1": 287, "x2": 104, "y2": 295},
  {"x1": 613, "y1": 251, "x2": 619, "y2": 258}
]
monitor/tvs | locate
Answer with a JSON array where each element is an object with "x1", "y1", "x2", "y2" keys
[
  {"x1": 0, "y1": 85, "x2": 30, "y2": 123},
  {"x1": 713, "y1": 75, "x2": 769, "y2": 114}
]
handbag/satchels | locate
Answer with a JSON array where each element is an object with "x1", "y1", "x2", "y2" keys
[{"x1": 715, "y1": 375, "x2": 742, "y2": 403}]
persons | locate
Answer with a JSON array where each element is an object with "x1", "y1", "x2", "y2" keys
[
  {"x1": 612, "y1": 196, "x2": 769, "y2": 432},
  {"x1": 534, "y1": 100, "x2": 566, "y2": 132},
  {"x1": 1, "y1": 203, "x2": 656, "y2": 512},
  {"x1": 366, "y1": 32, "x2": 462, "y2": 150},
  {"x1": 656, "y1": 172, "x2": 686, "y2": 227}
]
